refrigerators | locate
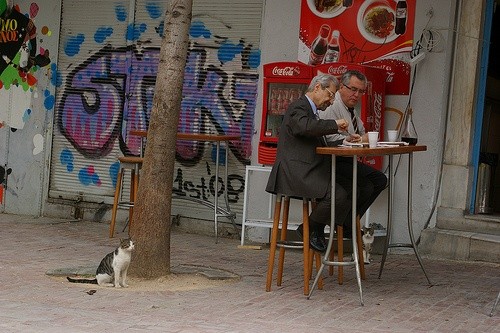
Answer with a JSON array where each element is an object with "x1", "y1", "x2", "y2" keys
[{"x1": 257, "y1": 61, "x2": 386, "y2": 174}]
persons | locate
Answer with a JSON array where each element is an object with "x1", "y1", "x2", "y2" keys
[
  {"x1": 264, "y1": 74, "x2": 350, "y2": 252},
  {"x1": 318, "y1": 70, "x2": 386, "y2": 238}
]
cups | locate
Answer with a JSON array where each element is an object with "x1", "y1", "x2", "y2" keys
[
  {"x1": 387, "y1": 131, "x2": 398, "y2": 142},
  {"x1": 368, "y1": 132, "x2": 379, "y2": 147}
]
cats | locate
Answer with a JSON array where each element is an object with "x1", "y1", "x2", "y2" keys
[{"x1": 67, "y1": 237, "x2": 135, "y2": 288}]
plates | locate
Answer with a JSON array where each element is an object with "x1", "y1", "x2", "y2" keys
[
  {"x1": 356, "y1": 0, "x2": 400, "y2": 44},
  {"x1": 306, "y1": 0, "x2": 347, "y2": 18}
]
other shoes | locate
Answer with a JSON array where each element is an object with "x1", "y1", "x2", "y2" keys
[{"x1": 336, "y1": 226, "x2": 365, "y2": 239}]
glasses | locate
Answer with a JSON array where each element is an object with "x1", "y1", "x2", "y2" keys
[
  {"x1": 343, "y1": 84, "x2": 366, "y2": 96},
  {"x1": 325, "y1": 87, "x2": 336, "y2": 101}
]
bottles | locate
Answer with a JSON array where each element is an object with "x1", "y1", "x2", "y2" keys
[
  {"x1": 401, "y1": 108, "x2": 418, "y2": 145},
  {"x1": 395, "y1": 0, "x2": 408, "y2": 36},
  {"x1": 324, "y1": 30, "x2": 340, "y2": 64},
  {"x1": 309, "y1": 24, "x2": 331, "y2": 65}
]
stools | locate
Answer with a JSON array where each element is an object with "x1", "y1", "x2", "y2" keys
[
  {"x1": 265, "y1": 194, "x2": 366, "y2": 295},
  {"x1": 109, "y1": 156, "x2": 143, "y2": 237}
]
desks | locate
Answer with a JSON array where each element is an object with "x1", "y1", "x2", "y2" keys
[
  {"x1": 128, "y1": 130, "x2": 241, "y2": 244},
  {"x1": 306, "y1": 144, "x2": 432, "y2": 304}
]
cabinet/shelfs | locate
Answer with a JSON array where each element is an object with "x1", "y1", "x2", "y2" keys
[{"x1": 241, "y1": 166, "x2": 393, "y2": 263}]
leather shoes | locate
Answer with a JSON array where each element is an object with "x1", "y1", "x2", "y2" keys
[{"x1": 296, "y1": 223, "x2": 326, "y2": 253}]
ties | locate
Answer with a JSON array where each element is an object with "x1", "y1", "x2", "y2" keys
[
  {"x1": 347, "y1": 107, "x2": 359, "y2": 134},
  {"x1": 315, "y1": 111, "x2": 328, "y2": 147}
]
